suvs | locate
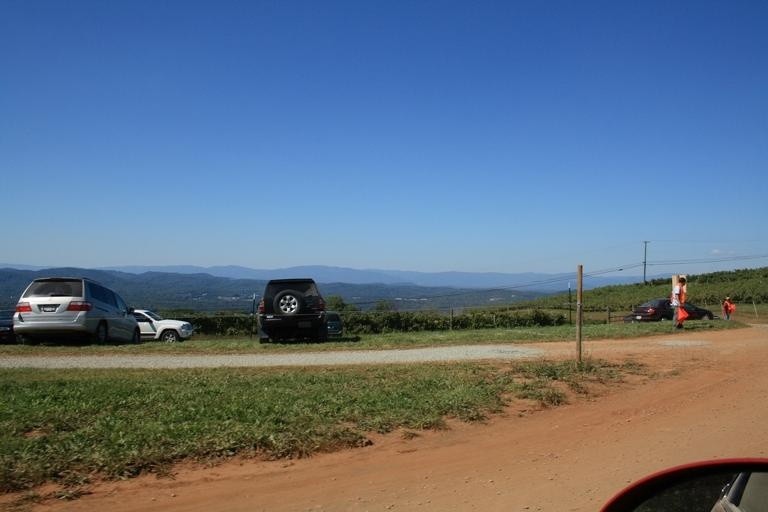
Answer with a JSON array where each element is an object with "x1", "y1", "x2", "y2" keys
[
  {"x1": 130, "y1": 309, "x2": 194, "y2": 343},
  {"x1": 256, "y1": 276, "x2": 330, "y2": 344}
]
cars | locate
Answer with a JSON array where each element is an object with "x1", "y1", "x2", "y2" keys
[
  {"x1": 324, "y1": 310, "x2": 343, "y2": 340},
  {"x1": 630, "y1": 297, "x2": 714, "y2": 322},
  {"x1": 0, "y1": 307, "x2": 21, "y2": 343}
]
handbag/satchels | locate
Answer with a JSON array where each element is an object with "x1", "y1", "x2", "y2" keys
[{"x1": 677, "y1": 306, "x2": 689, "y2": 322}]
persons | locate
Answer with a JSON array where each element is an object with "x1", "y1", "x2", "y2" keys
[
  {"x1": 722, "y1": 297, "x2": 733, "y2": 320},
  {"x1": 670, "y1": 277, "x2": 688, "y2": 331}
]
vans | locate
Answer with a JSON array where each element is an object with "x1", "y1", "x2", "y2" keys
[{"x1": 11, "y1": 273, "x2": 142, "y2": 346}]
